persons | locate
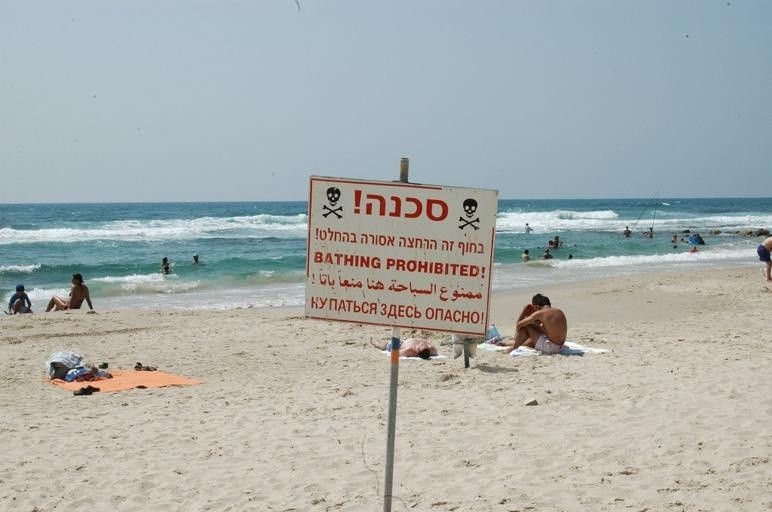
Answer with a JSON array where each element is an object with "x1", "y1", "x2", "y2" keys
[
  {"x1": 45, "y1": 272, "x2": 94, "y2": 313},
  {"x1": 8, "y1": 283, "x2": 34, "y2": 314},
  {"x1": 622, "y1": 225, "x2": 706, "y2": 253},
  {"x1": 368, "y1": 335, "x2": 439, "y2": 360},
  {"x1": 161, "y1": 256, "x2": 169, "y2": 268},
  {"x1": 756, "y1": 234, "x2": 771, "y2": 282},
  {"x1": 162, "y1": 265, "x2": 170, "y2": 273},
  {"x1": 498, "y1": 295, "x2": 568, "y2": 355},
  {"x1": 498, "y1": 292, "x2": 543, "y2": 347},
  {"x1": 191, "y1": 254, "x2": 203, "y2": 265},
  {"x1": 520, "y1": 221, "x2": 577, "y2": 262}
]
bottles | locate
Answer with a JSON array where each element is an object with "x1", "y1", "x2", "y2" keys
[{"x1": 488, "y1": 323, "x2": 497, "y2": 339}]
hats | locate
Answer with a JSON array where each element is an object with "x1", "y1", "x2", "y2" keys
[{"x1": 16, "y1": 285, "x2": 25, "y2": 290}]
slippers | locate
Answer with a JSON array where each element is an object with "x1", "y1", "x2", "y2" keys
[{"x1": 73, "y1": 385, "x2": 100, "y2": 395}]
